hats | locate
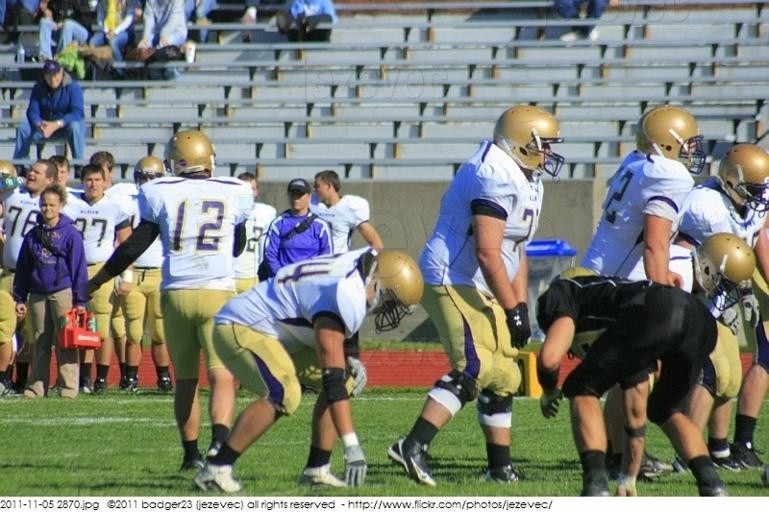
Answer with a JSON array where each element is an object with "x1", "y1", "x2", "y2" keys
[
  {"x1": 44, "y1": 61, "x2": 60, "y2": 75},
  {"x1": 289, "y1": 179, "x2": 312, "y2": 195}
]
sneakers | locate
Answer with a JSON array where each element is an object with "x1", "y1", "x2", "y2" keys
[
  {"x1": 485, "y1": 464, "x2": 528, "y2": 485},
  {"x1": 195, "y1": 461, "x2": 244, "y2": 493},
  {"x1": 652, "y1": 454, "x2": 673, "y2": 473},
  {"x1": 388, "y1": 435, "x2": 437, "y2": 487},
  {"x1": 180, "y1": 458, "x2": 206, "y2": 472},
  {"x1": 701, "y1": 480, "x2": 728, "y2": 497},
  {"x1": 117, "y1": 379, "x2": 138, "y2": 395},
  {"x1": 674, "y1": 456, "x2": 691, "y2": 473},
  {"x1": 581, "y1": 488, "x2": 612, "y2": 496},
  {"x1": 95, "y1": 378, "x2": 109, "y2": 396},
  {"x1": 709, "y1": 449, "x2": 741, "y2": 474},
  {"x1": 612, "y1": 451, "x2": 665, "y2": 481},
  {"x1": 298, "y1": 464, "x2": 349, "y2": 490},
  {"x1": 157, "y1": 373, "x2": 176, "y2": 397},
  {"x1": 82, "y1": 378, "x2": 93, "y2": 398},
  {"x1": 207, "y1": 441, "x2": 224, "y2": 456},
  {"x1": 734, "y1": 439, "x2": 764, "y2": 469}
]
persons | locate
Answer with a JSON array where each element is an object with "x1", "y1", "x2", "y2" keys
[
  {"x1": 386, "y1": 93, "x2": 564, "y2": 486},
  {"x1": 6, "y1": 60, "x2": 87, "y2": 176},
  {"x1": 551, "y1": 0, "x2": 620, "y2": 42},
  {"x1": 0, "y1": 0, "x2": 217, "y2": 78}
]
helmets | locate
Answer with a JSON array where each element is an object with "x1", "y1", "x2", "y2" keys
[
  {"x1": 559, "y1": 268, "x2": 595, "y2": 278},
  {"x1": 134, "y1": 157, "x2": 165, "y2": 180},
  {"x1": 370, "y1": 253, "x2": 425, "y2": 334},
  {"x1": 494, "y1": 106, "x2": 565, "y2": 175},
  {"x1": 699, "y1": 233, "x2": 758, "y2": 312},
  {"x1": 0, "y1": 160, "x2": 18, "y2": 180},
  {"x1": 718, "y1": 144, "x2": 769, "y2": 213},
  {"x1": 165, "y1": 130, "x2": 215, "y2": 175},
  {"x1": 637, "y1": 106, "x2": 709, "y2": 175}
]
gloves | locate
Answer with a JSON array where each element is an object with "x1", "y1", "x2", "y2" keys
[
  {"x1": 506, "y1": 305, "x2": 530, "y2": 351},
  {"x1": 541, "y1": 388, "x2": 563, "y2": 419},
  {"x1": 519, "y1": 303, "x2": 533, "y2": 337},
  {"x1": 84, "y1": 271, "x2": 111, "y2": 296},
  {"x1": 743, "y1": 291, "x2": 761, "y2": 328},
  {"x1": 722, "y1": 308, "x2": 742, "y2": 336},
  {"x1": 345, "y1": 445, "x2": 367, "y2": 487},
  {"x1": 347, "y1": 356, "x2": 368, "y2": 397}
]
